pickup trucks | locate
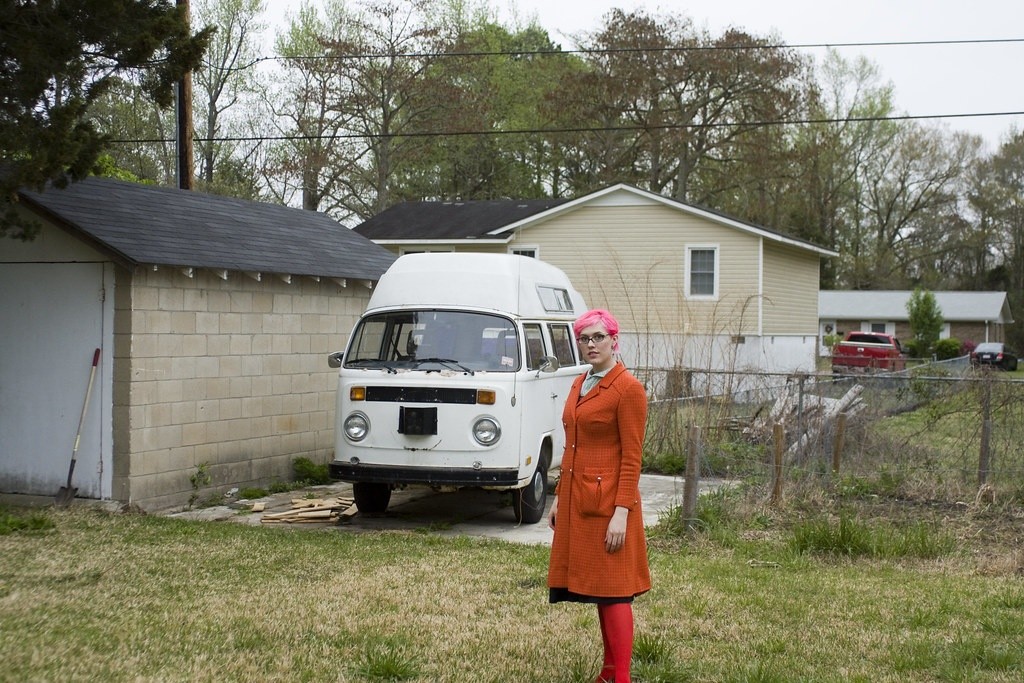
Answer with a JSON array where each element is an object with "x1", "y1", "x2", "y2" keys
[{"x1": 831, "y1": 332, "x2": 907, "y2": 386}]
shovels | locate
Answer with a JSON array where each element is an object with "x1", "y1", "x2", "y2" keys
[{"x1": 53, "y1": 347, "x2": 101, "y2": 510}]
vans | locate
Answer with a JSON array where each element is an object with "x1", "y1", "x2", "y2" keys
[{"x1": 325, "y1": 249, "x2": 593, "y2": 526}]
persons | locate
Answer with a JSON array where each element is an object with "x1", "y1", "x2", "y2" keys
[{"x1": 547, "y1": 310, "x2": 651, "y2": 683}]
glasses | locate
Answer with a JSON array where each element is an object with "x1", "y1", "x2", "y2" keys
[{"x1": 576, "y1": 334, "x2": 610, "y2": 344}]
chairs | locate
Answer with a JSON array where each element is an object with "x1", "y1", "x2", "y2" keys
[
  {"x1": 395, "y1": 326, "x2": 454, "y2": 360},
  {"x1": 486, "y1": 330, "x2": 534, "y2": 369}
]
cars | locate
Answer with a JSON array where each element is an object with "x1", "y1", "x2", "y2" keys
[{"x1": 969, "y1": 340, "x2": 1019, "y2": 372}]
